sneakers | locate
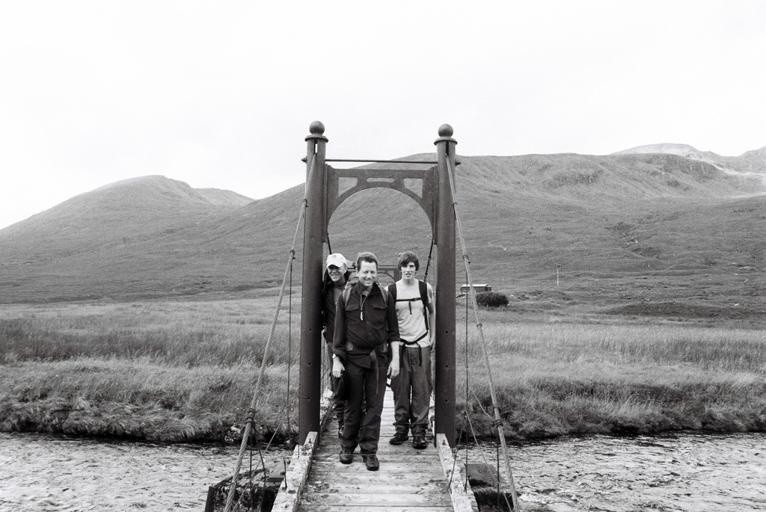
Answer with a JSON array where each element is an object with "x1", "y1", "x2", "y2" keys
[
  {"x1": 340, "y1": 449, "x2": 355, "y2": 463},
  {"x1": 389, "y1": 434, "x2": 408, "y2": 446},
  {"x1": 362, "y1": 454, "x2": 379, "y2": 470},
  {"x1": 412, "y1": 436, "x2": 428, "y2": 449}
]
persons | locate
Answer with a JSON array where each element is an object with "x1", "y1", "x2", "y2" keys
[
  {"x1": 331, "y1": 252, "x2": 400, "y2": 471},
  {"x1": 323, "y1": 253, "x2": 356, "y2": 438},
  {"x1": 385, "y1": 251, "x2": 436, "y2": 449}
]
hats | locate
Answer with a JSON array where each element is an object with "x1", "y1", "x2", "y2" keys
[{"x1": 326, "y1": 253, "x2": 346, "y2": 267}]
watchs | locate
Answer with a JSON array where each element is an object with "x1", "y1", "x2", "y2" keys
[{"x1": 332, "y1": 353, "x2": 337, "y2": 359}]
